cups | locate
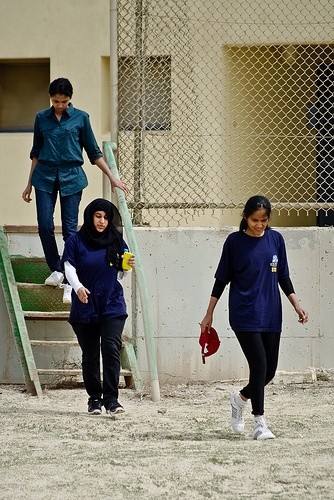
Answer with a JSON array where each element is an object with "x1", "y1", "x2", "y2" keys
[{"x1": 122, "y1": 252, "x2": 133, "y2": 270}]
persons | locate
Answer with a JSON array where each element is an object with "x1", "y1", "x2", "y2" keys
[
  {"x1": 22, "y1": 78, "x2": 131, "y2": 305},
  {"x1": 201, "y1": 196, "x2": 309, "y2": 440},
  {"x1": 58, "y1": 199, "x2": 135, "y2": 412}
]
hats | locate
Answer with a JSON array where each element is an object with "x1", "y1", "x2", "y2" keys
[{"x1": 198, "y1": 322, "x2": 220, "y2": 364}]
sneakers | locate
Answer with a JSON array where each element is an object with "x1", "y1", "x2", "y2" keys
[
  {"x1": 59, "y1": 284, "x2": 72, "y2": 304},
  {"x1": 45, "y1": 271, "x2": 64, "y2": 286},
  {"x1": 230, "y1": 392, "x2": 245, "y2": 435},
  {"x1": 251, "y1": 420, "x2": 275, "y2": 442}
]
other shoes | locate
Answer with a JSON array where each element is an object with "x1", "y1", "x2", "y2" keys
[
  {"x1": 87, "y1": 397, "x2": 103, "y2": 415},
  {"x1": 104, "y1": 400, "x2": 126, "y2": 415}
]
